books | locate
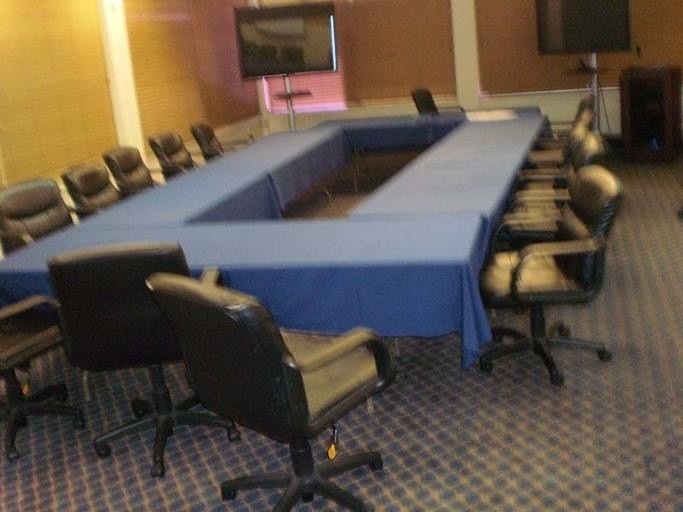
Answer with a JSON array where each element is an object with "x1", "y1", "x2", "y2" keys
[{"x1": 465, "y1": 109, "x2": 518, "y2": 123}]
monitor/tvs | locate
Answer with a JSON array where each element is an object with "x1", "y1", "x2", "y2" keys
[
  {"x1": 233, "y1": 1, "x2": 338, "y2": 80},
  {"x1": 535, "y1": 0, "x2": 630, "y2": 55}
]
